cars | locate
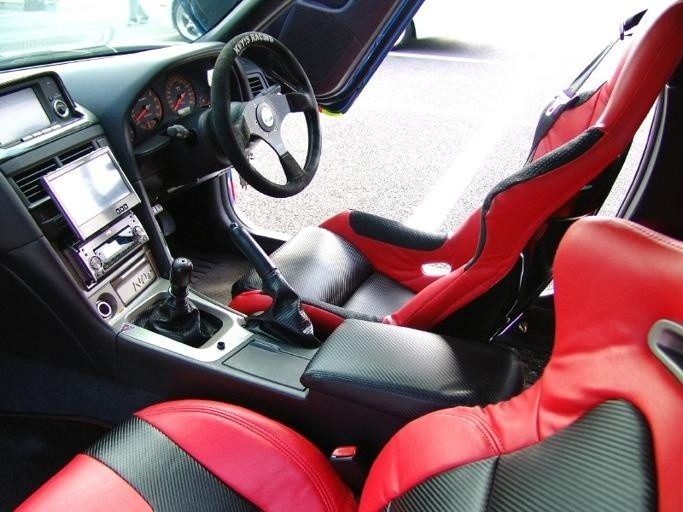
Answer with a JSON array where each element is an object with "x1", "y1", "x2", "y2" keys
[{"x1": 138, "y1": 0, "x2": 462, "y2": 51}]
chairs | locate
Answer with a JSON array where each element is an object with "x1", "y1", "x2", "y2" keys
[
  {"x1": 229, "y1": 0, "x2": 683, "y2": 330},
  {"x1": 14, "y1": 214, "x2": 683, "y2": 512}
]
